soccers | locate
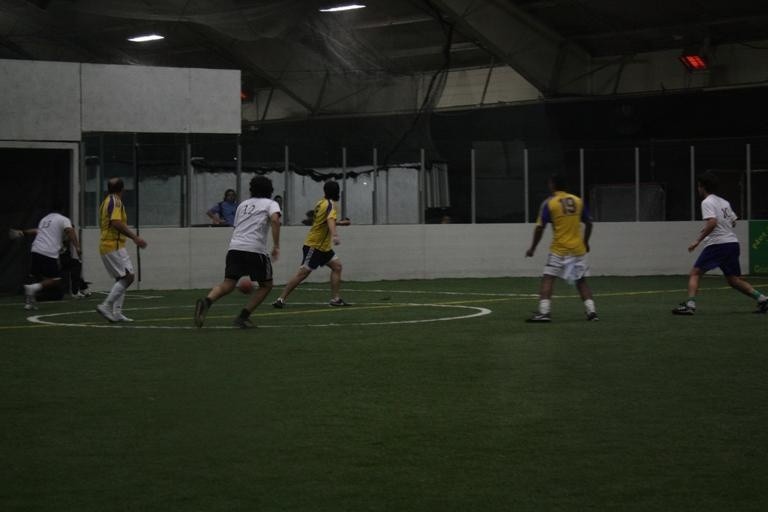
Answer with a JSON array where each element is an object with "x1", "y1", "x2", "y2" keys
[{"x1": 238, "y1": 276, "x2": 252, "y2": 293}]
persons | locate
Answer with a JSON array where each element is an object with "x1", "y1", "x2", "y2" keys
[
  {"x1": 272, "y1": 182, "x2": 351, "y2": 310},
  {"x1": 96, "y1": 178, "x2": 146, "y2": 322},
  {"x1": 9, "y1": 225, "x2": 91, "y2": 297},
  {"x1": 524, "y1": 175, "x2": 597, "y2": 321},
  {"x1": 302, "y1": 210, "x2": 314, "y2": 225},
  {"x1": 194, "y1": 177, "x2": 281, "y2": 329},
  {"x1": 206, "y1": 189, "x2": 237, "y2": 227},
  {"x1": 274, "y1": 196, "x2": 285, "y2": 227},
  {"x1": 441, "y1": 215, "x2": 451, "y2": 223},
  {"x1": 23, "y1": 202, "x2": 73, "y2": 310},
  {"x1": 671, "y1": 175, "x2": 768, "y2": 314}
]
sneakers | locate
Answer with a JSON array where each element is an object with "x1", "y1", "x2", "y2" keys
[
  {"x1": 671, "y1": 302, "x2": 697, "y2": 316},
  {"x1": 329, "y1": 298, "x2": 352, "y2": 308},
  {"x1": 193, "y1": 297, "x2": 210, "y2": 329},
  {"x1": 272, "y1": 299, "x2": 285, "y2": 309},
  {"x1": 71, "y1": 288, "x2": 92, "y2": 298},
  {"x1": 24, "y1": 284, "x2": 40, "y2": 311},
  {"x1": 584, "y1": 311, "x2": 599, "y2": 322},
  {"x1": 525, "y1": 311, "x2": 552, "y2": 323},
  {"x1": 232, "y1": 316, "x2": 256, "y2": 329},
  {"x1": 752, "y1": 298, "x2": 768, "y2": 313},
  {"x1": 96, "y1": 304, "x2": 135, "y2": 323}
]
